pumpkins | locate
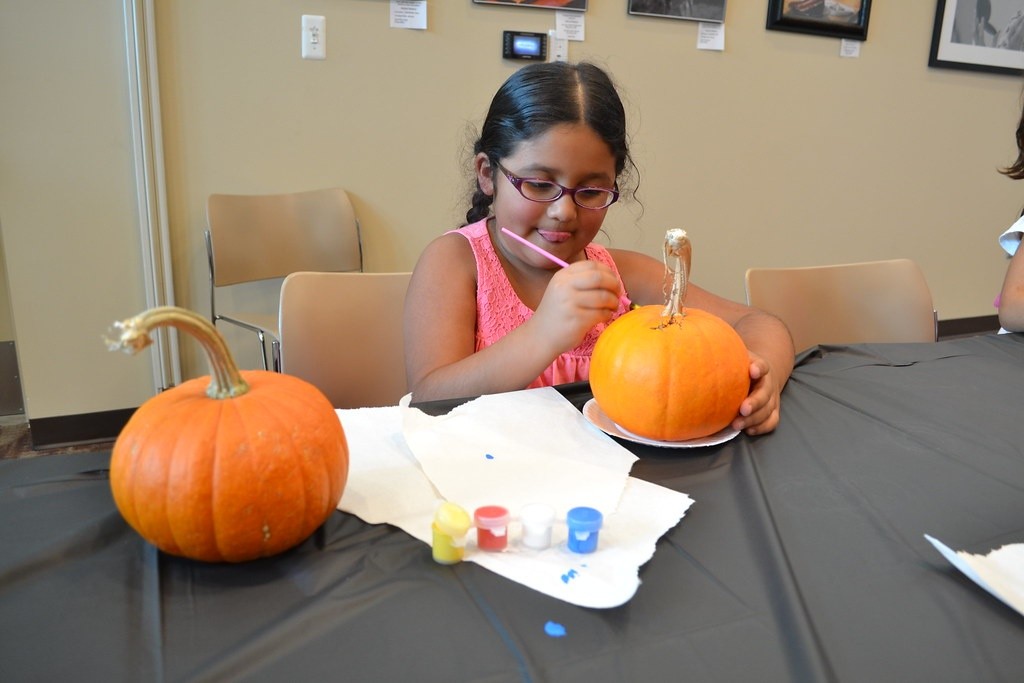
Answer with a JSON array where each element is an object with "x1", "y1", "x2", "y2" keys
[
  {"x1": 588, "y1": 228, "x2": 751, "y2": 445},
  {"x1": 109, "y1": 306, "x2": 349, "y2": 562}
]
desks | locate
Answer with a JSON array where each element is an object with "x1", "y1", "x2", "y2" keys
[{"x1": 0, "y1": 334, "x2": 1024, "y2": 683}]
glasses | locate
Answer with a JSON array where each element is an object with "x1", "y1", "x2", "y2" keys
[{"x1": 493, "y1": 159, "x2": 619, "y2": 210}]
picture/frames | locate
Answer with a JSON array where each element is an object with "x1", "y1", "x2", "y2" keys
[{"x1": 474, "y1": 0, "x2": 1024, "y2": 76}]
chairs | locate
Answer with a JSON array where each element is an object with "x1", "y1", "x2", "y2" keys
[
  {"x1": 744, "y1": 258, "x2": 936, "y2": 357},
  {"x1": 204, "y1": 189, "x2": 411, "y2": 410}
]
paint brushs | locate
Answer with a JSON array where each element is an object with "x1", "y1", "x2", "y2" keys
[{"x1": 500, "y1": 228, "x2": 640, "y2": 310}]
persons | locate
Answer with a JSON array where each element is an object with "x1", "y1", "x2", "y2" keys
[
  {"x1": 998, "y1": 95, "x2": 1024, "y2": 332},
  {"x1": 400, "y1": 60, "x2": 795, "y2": 436}
]
